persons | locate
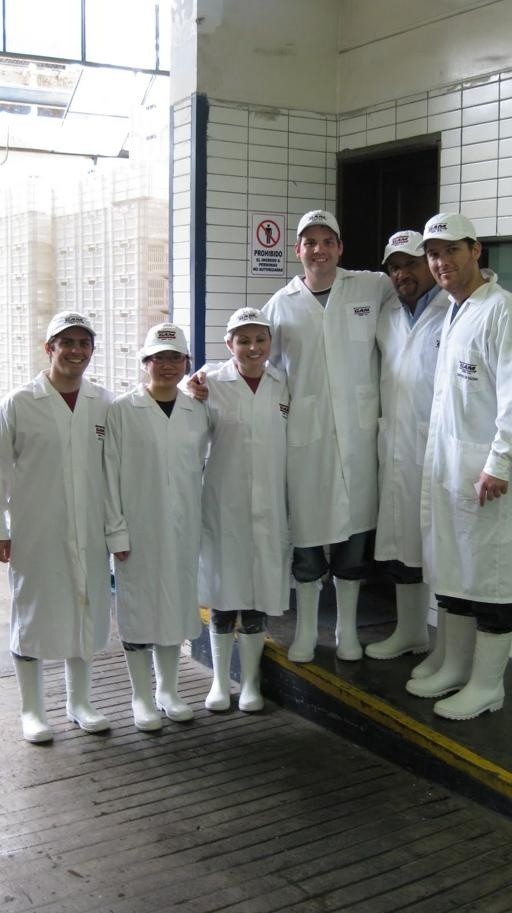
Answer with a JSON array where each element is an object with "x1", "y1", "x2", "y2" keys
[
  {"x1": 102, "y1": 322, "x2": 210, "y2": 733},
  {"x1": 175, "y1": 304, "x2": 296, "y2": 714},
  {"x1": 360, "y1": 229, "x2": 454, "y2": 677},
  {"x1": 0, "y1": 310, "x2": 115, "y2": 744},
  {"x1": 403, "y1": 210, "x2": 512, "y2": 719},
  {"x1": 261, "y1": 207, "x2": 389, "y2": 663}
]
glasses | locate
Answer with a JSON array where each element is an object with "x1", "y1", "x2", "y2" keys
[{"x1": 149, "y1": 355, "x2": 186, "y2": 364}]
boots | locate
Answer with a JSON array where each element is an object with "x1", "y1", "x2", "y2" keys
[
  {"x1": 286, "y1": 578, "x2": 322, "y2": 663},
  {"x1": 64, "y1": 657, "x2": 110, "y2": 733},
  {"x1": 152, "y1": 644, "x2": 194, "y2": 721},
  {"x1": 365, "y1": 582, "x2": 511, "y2": 720},
  {"x1": 236, "y1": 628, "x2": 266, "y2": 711},
  {"x1": 333, "y1": 576, "x2": 363, "y2": 661},
  {"x1": 14, "y1": 657, "x2": 53, "y2": 742},
  {"x1": 204, "y1": 631, "x2": 234, "y2": 711},
  {"x1": 124, "y1": 647, "x2": 162, "y2": 731}
]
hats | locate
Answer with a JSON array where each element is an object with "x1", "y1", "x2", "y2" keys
[
  {"x1": 296, "y1": 210, "x2": 341, "y2": 242},
  {"x1": 139, "y1": 322, "x2": 190, "y2": 362},
  {"x1": 45, "y1": 311, "x2": 96, "y2": 345},
  {"x1": 381, "y1": 229, "x2": 425, "y2": 265},
  {"x1": 226, "y1": 307, "x2": 271, "y2": 333},
  {"x1": 415, "y1": 212, "x2": 477, "y2": 252}
]
